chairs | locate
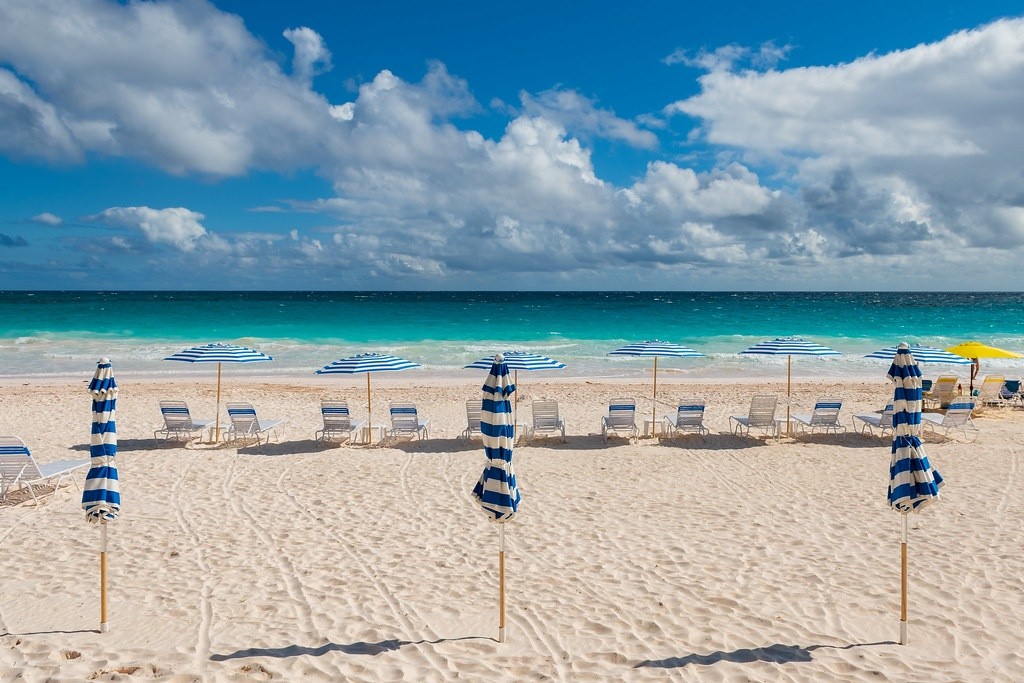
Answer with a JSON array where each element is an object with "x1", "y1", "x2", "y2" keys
[
  {"x1": 222, "y1": 400, "x2": 287, "y2": 448},
  {"x1": 662, "y1": 398, "x2": 709, "y2": 443},
  {"x1": 600, "y1": 397, "x2": 640, "y2": 444},
  {"x1": 918, "y1": 375, "x2": 1024, "y2": 443},
  {"x1": 852, "y1": 398, "x2": 895, "y2": 445},
  {"x1": 461, "y1": 400, "x2": 482, "y2": 440},
  {"x1": 529, "y1": 399, "x2": 565, "y2": 442},
  {"x1": 0, "y1": 435, "x2": 91, "y2": 508},
  {"x1": 790, "y1": 394, "x2": 847, "y2": 442},
  {"x1": 315, "y1": 400, "x2": 368, "y2": 448},
  {"x1": 728, "y1": 395, "x2": 778, "y2": 443},
  {"x1": 389, "y1": 401, "x2": 432, "y2": 450},
  {"x1": 153, "y1": 400, "x2": 217, "y2": 450}
]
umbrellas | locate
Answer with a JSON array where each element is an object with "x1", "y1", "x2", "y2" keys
[
  {"x1": 77, "y1": 357, "x2": 123, "y2": 632},
  {"x1": 944, "y1": 338, "x2": 1024, "y2": 397},
  {"x1": 472, "y1": 353, "x2": 524, "y2": 644},
  {"x1": 737, "y1": 334, "x2": 844, "y2": 432},
  {"x1": 861, "y1": 337, "x2": 977, "y2": 370},
  {"x1": 607, "y1": 337, "x2": 707, "y2": 438},
  {"x1": 164, "y1": 343, "x2": 275, "y2": 446},
  {"x1": 885, "y1": 340, "x2": 946, "y2": 644},
  {"x1": 316, "y1": 351, "x2": 422, "y2": 445},
  {"x1": 464, "y1": 349, "x2": 568, "y2": 443}
]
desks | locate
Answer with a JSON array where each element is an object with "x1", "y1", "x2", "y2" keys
[
  {"x1": 362, "y1": 421, "x2": 386, "y2": 444},
  {"x1": 644, "y1": 418, "x2": 665, "y2": 435},
  {"x1": 513, "y1": 418, "x2": 528, "y2": 439},
  {"x1": 208, "y1": 422, "x2": 232, "y2": 445},
  {"x1": 775, "y1": 417, "x2": 795, "y2": 435}
]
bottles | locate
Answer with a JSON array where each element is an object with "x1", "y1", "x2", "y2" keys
[
  {"x1": 958, "y1": 383, "x2": 962, "y2": 396},
  {"x1": 973, "y1": 390, "x2": 978, "y2": 396}
]
